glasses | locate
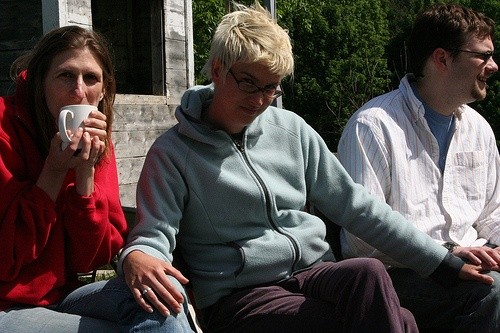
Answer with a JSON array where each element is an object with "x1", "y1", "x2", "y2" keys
[
  {"x1": 445, "y1": 48, "x2": 497, "y2": 64},
  {"x1": 224, "y1": 60, "x2": 285, "y2": 99}
]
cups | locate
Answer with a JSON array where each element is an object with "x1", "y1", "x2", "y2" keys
[{"x1": 57, "y1": 104, "x2": 99, "y2": 153}]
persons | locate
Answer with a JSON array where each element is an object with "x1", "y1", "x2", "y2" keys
[
  {"x1": 338, "y1": 6, "x2": 500, "y2": 333},
  {"x1": 115, "y1": 9, "x2": 500, "y2": 333},
  {"x1": 0, "y1": 26, "x2": 202, "y2": 332}
]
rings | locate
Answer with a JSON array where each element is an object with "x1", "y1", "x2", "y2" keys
[{"x1": 143, "y1": 287, "x2": 151, "y2": 294}]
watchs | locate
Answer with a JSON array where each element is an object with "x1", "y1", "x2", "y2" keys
[{"x1": 443, "y1": 241, "x2": 460, "y2": 252}]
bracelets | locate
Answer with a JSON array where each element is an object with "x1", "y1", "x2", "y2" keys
[{"x1": 431, "y1": 252, "x2": 464, "y2": 285}]
